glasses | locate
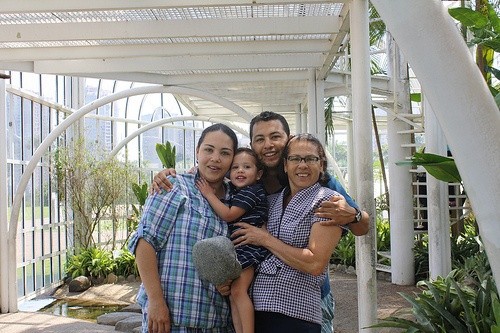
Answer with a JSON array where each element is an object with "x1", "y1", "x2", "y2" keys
[{"x1": 287, "y1": 155, "x2": 321, "y2": 164}]
